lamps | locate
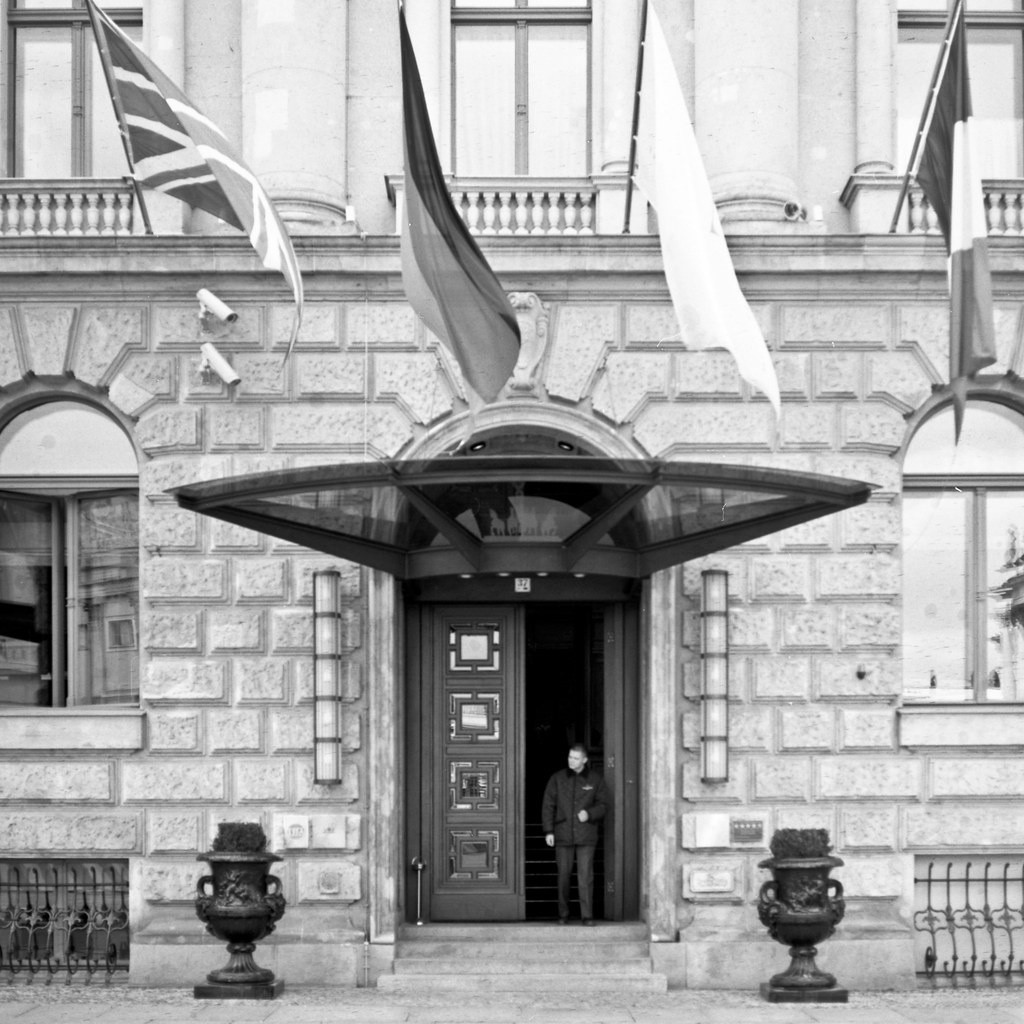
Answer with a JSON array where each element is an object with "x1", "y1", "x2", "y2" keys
[
  {"x1": 313, "y1": 570, "x2": 343, "y2": 784},
  {"x1": 701, "y1": 569, "x2": 729, "y2": 783}
]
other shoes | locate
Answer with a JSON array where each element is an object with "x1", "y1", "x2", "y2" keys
[
  {"x1": 560, "y1": 916, "x2": 566, "y2": 923},
  {"x1": 583, "y1": 917, "x2": 595, "y2": 926}
]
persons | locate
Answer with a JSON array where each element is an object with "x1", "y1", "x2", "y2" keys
[{"x1": 542, "y1": 746, "x2": 610, "y2": 927}]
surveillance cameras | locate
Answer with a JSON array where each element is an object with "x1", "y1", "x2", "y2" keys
[
  {"x1": 200, "y1": 342, "x2": 243, "y2": 385},
  {"x1": 195, "y1": 287, "x2": 238, "y2": 325}
]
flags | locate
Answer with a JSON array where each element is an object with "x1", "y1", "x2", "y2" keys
[
  {"x1": 394, "y1": 0, "x2": 521, "y2": 454},
  {"x1": 84, "y1": 0, "x2": 304, "y2": 371},
  {"x1": 910, "y1": 0, "x2": 996, "y2": 447},
  {"x1": 631, "y1": 0, "x2": 784, "y2": 456}
]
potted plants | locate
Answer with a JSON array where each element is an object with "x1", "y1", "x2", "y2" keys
[
  {"x1": 758, "y1": 828, "x2": 848, "y2": 1004},
  {"x1": 194, "y1": 823, "x2": 287, "y2": 1001}
]
